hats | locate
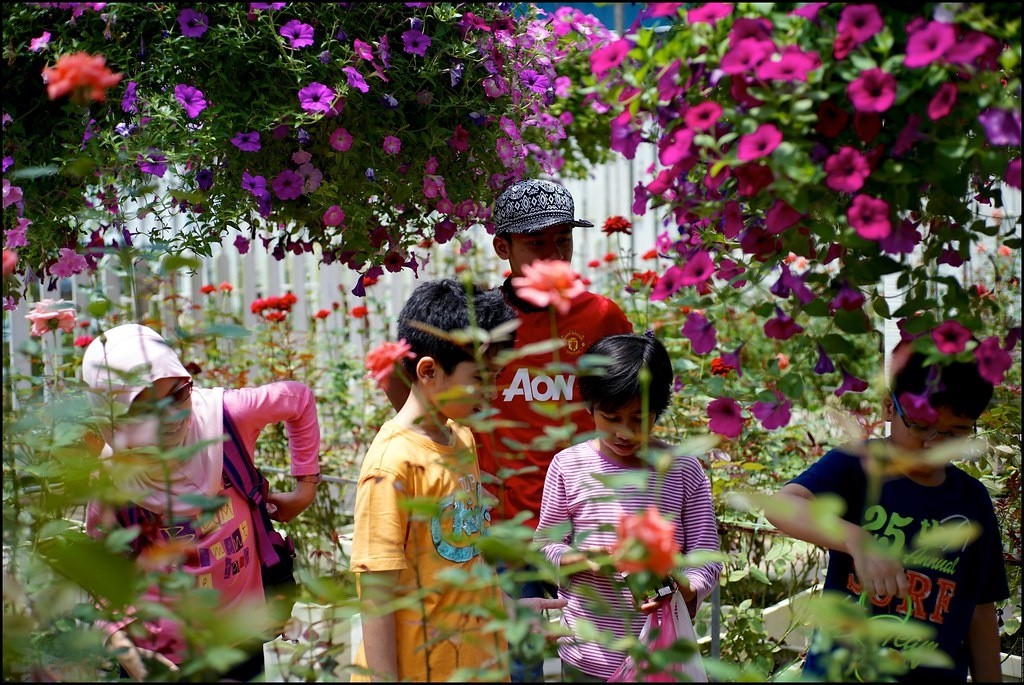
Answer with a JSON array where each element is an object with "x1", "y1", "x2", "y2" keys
[{"x1": 493, "y1": 178, "x2": 596, "y2": 234}]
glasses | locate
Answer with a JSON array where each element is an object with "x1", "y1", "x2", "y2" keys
[
  {"x1": 889, "y1": 389, "x2": 978, "y2": 442},
  {"x1": 136, "y1": 381, "x2": 194, "y2": 418}
]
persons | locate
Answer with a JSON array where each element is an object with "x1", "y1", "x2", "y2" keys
[
  {"x1": 384, "y1": 178, "x2": 639, "y2": 685},
  {"x1": 533, "y1": 332, "x2": 726, "y2": 685},
  {"x1": 762, "y1": 328, "x2": 1011, "y2": 683},
  {"x1": 350, "y1": 282, "x2": 521, "y2": 685},
  {"x1": 80, "y1": 324, "x2": 321, "y2": 685}
]
